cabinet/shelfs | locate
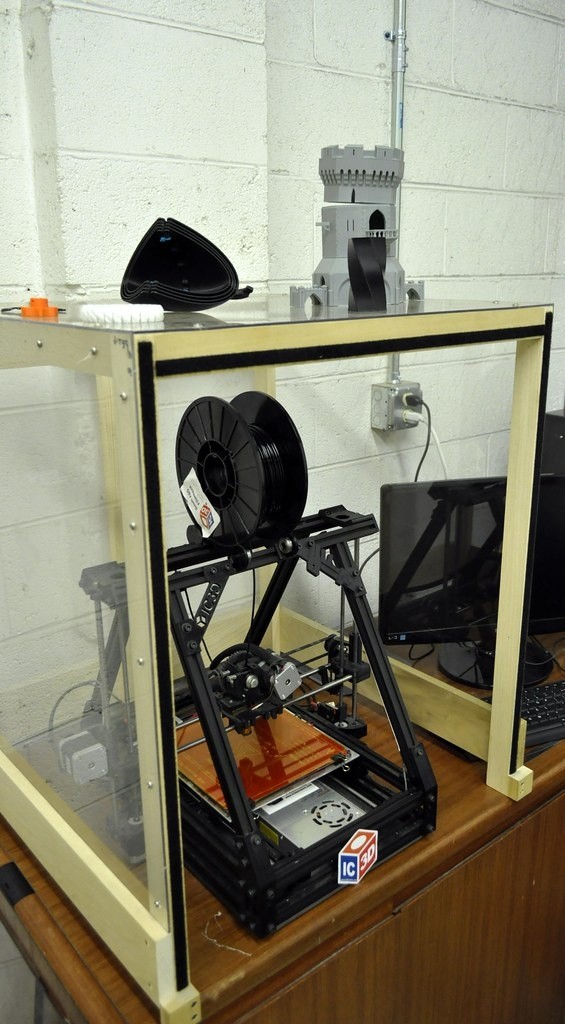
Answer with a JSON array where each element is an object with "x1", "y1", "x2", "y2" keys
[{"x1": 1, "y1": 616, "x2": 565, "y2": 1024}]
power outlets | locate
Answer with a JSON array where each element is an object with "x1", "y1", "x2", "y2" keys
[{"x1": 370, "y1": 380, "x2": 423, "y2": 430}]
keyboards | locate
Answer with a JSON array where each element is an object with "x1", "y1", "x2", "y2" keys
[{"x1": 479, "y1": 678, "x2": 565, "y2": 747}]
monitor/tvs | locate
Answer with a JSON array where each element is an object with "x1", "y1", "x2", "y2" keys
[{"x1": 379, "y1": 471, "x2": 565, "y2": 689}]
